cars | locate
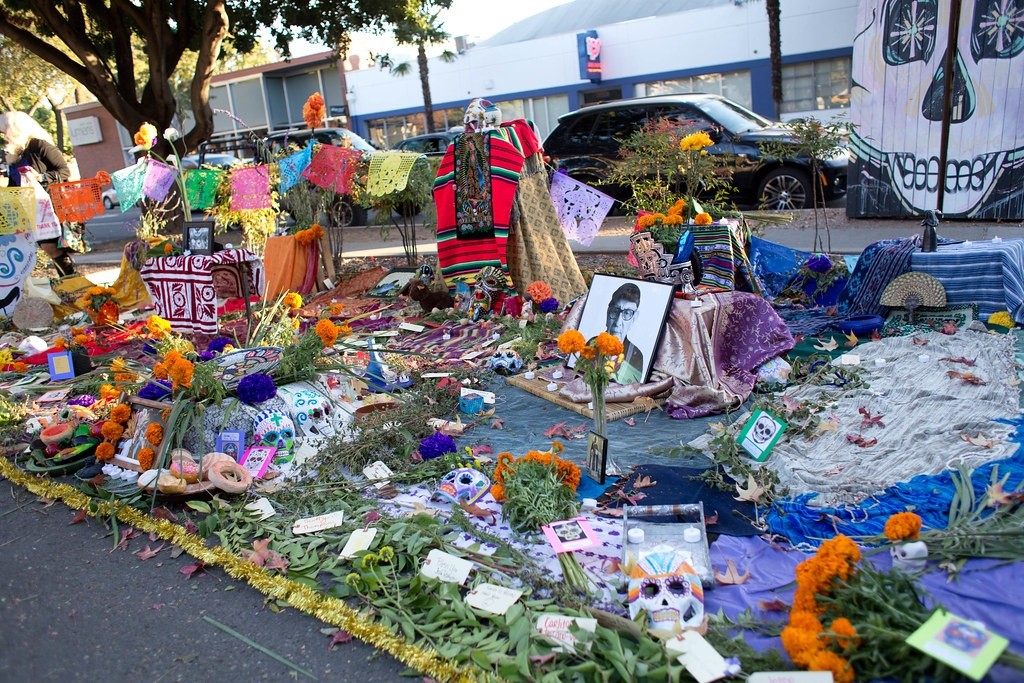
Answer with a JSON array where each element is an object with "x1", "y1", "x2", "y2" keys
[
  {"x1": 382, "y1": 124, "x2": 467, "y2": 218},
  {"x1": 103, "y1": 121, "x2": 467, "y2": 229}
]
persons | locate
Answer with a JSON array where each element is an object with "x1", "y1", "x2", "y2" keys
[
  {"x1": 579, "y1": 283, "x2": 643, "y2": 385},
  {"x1": 0, "y1": 111, "x2": 75, "y2": 278}
]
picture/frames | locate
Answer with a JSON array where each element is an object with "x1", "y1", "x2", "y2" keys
[
  {"x1": 183, "y1": 220, "x2": 214, "y2": 255},
  {"x1": 108, "y1": 397, "x2": 178, "y2": 473},
  {"x1": 587, "y1": 431, "x2": 608, "y2": 485},
  {"x1": 562, "y1": 271, "x2": 675, "y2": 382}
]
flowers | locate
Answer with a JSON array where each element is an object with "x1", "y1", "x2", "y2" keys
[
  {"x1": 782, "y1": 512, "x2": 1023, "y2": 682},
  {"x1": 54, "y1": 276, "x2": 433, "y2": 519},
  {"x1": 348, "y1": 546, "x2": 446, "y2": 661},
  {"x1": 525, "y1": 282, "x2": 558, "y2": 315},
  {"x1": 0, "y1": 346, "x2": 30, "y2": 380},
  {"x1": 300, "y1": 92, "x2": 325, "y2": 138},
  {"x1": 491, "y1": 443, "x2": 597, "y2": 594},
  {"x1": 289, "y1": 222, "x2": 326, "y2": 245},
  {"x1": 557, "y1": 329, "x2": 628, "y2": 432},
  {"x1": 592, "y1": 119, "x2": 795, "y2": 268},
  {"x1": 132, "y1": 120, "x2": 190, "y2": 219},
  {"x1": 988, "y1": 312, "x2": 1015, "y2": 330}
]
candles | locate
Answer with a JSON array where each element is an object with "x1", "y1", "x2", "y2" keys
[
  {"x1": 127, "y1": 471, "x2": 137, "y2": 481},
  {"x1": 110, "y1": 469, "x2": 123, "y2": 477},
  {"x1": 628, "y1": 523, "x2": 642, "y2": 546},
  {"x1": 684, "y1": 527, "x2": 702, "y2": 542},
  {"x1": 102, "y1": 462, "x2": 114, "y2": 474},
  {"x1": 121, "y1": 469, "x2": 129, "y2": 479},
  {"x1": 108, "y1": 466, "x2": 119, "y2": 474}
]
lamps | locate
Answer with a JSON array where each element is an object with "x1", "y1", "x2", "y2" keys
[{"x1": 455, "y1": 34, "x2": 468, "y2": 55}]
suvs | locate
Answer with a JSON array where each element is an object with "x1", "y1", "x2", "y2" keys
[{"x1": 540, "y1": 90, "x2": 849, "y2": 216}]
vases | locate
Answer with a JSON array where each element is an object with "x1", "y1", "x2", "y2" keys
[{"x1": 589, "y1": 382, "x2": 623, "y2": 476}]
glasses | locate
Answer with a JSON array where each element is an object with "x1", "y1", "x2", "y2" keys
[{"x1": 608, "y1": 306, "x2": 637, "y2": 321}]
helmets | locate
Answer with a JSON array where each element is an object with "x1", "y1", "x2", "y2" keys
[{"x1": 463, "y1": 99, "x2": 502, "y2": 133}]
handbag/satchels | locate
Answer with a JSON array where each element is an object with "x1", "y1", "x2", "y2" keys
[{"x1": 20, "y1": 171, "x2": 61, "y2": 242}]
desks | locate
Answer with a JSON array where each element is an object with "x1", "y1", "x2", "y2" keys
[{"x1": 141, "y1": 250, "x2": 257, "y2": 334}]
